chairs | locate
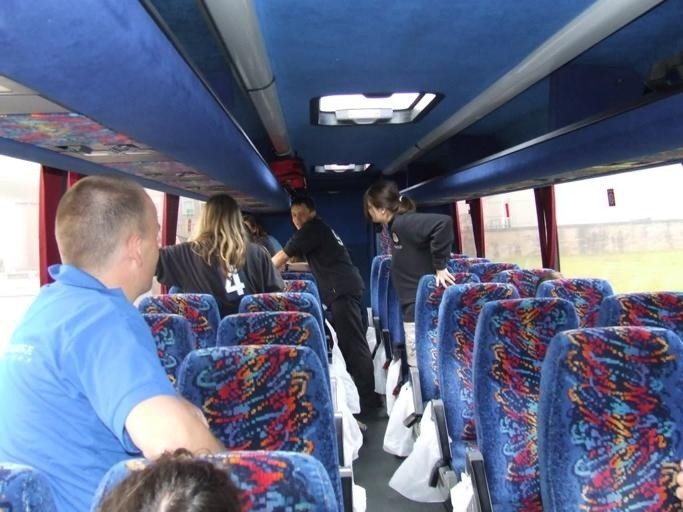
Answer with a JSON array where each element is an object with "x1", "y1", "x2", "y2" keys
[
  {"x1": 365, "y1": 251, "x2": 683, "y2": 511},
  {"x1": 0, "y1": 263, "x2": 355, "y2": 511}
]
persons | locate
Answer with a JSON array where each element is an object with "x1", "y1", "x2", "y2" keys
[
  {"x1": 95, "y1": 451, "x2": 242, "y2": 512},
  {"x1": 363, "y1": 179, "x2": 456, "y2": 365},
  {"x1": 154, "y1": 192, "x2": 285, "y2": 313},
  {"x1": 0, "y1": 174, "x2": 229, "y2": 512},
  {"x1": 272, "y1": 196, "x2": 386, "y2": 422}
]
